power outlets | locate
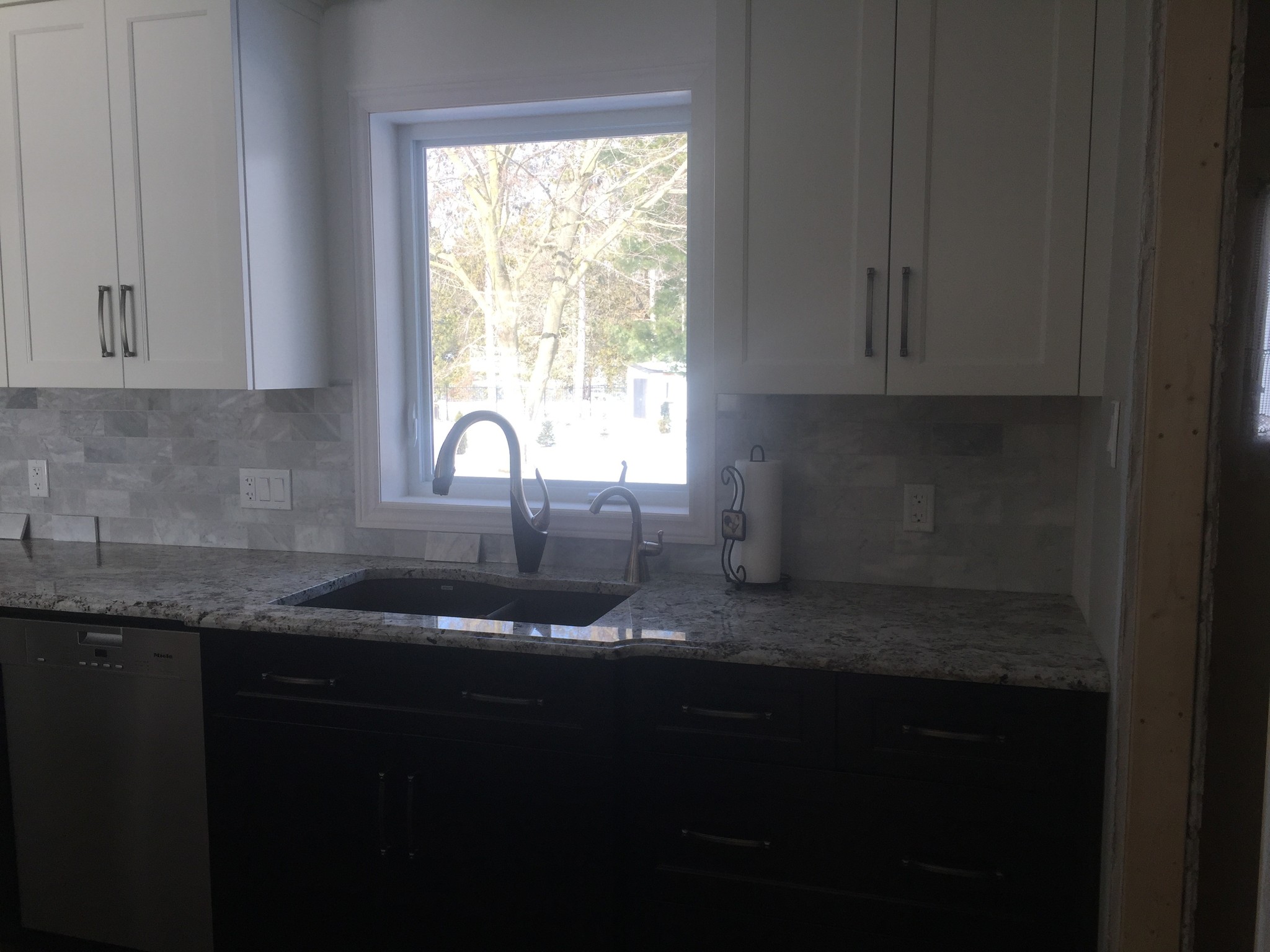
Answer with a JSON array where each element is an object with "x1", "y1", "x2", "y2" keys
[
  {"x1": 903, "y1": 482, "x2": 935, "y2": 533},
  {"x1": 238, "y1": 467, "x2": 292, "y2": 510},
  {"x1": 27, "y1": 459, "x2": 50, "y2": 497}
]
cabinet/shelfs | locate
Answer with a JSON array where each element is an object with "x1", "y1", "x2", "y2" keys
[
  {"x1": 0, "y1": 0, "x2": 332, "y2": 389},
  {"x1": 715, "y1": 0, "x2": 1100, "y2": 399},
  {"x1": 207, "y1": 630, "x2": 1107, "y2": 952}
]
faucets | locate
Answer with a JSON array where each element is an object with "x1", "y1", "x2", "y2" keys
[
  {"x1": 587, "y1": 484, "x2": 665, "y2": 583},
  {"x1": 431, "y1": 408, "x2": 554, "y2": 577}
]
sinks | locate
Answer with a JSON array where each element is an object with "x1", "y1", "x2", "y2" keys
[{"x1": 263, "y1": 565, "x2": 645, "y2": 629}]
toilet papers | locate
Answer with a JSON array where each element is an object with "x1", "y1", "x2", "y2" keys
[{"x1": 729, "y1": 459, "x2": 784, "y2": 584}]
